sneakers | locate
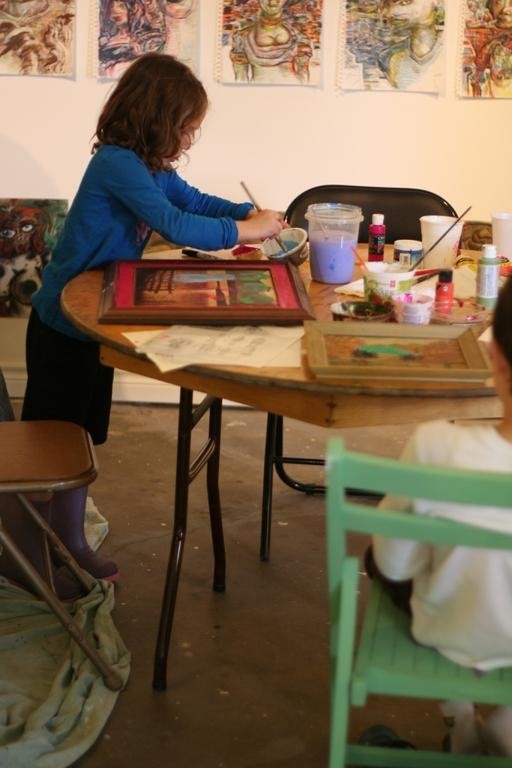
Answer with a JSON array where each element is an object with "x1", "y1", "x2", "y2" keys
[{"x1": 361, "y1": 724, "x2": 416, "y2": 749}]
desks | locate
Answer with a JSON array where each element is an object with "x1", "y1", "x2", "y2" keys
[{"x1": 60, "y1": 242, "x2": 511, "y2": 690}]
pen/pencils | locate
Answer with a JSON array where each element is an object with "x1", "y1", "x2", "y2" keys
[{"x1": 182, "y1": 250, "x2": 219, "y2": 260}]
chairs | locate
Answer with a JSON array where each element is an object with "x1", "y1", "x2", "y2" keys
[
  {"x1": 259, "y1": 184, "x2": 461, "y2": 561},
  {"x1": 0, "y1": 420, "x2": 125, "y2": 692},
  {"x1": 325, "y1": 436, "x2": 512, "y2": 767}
]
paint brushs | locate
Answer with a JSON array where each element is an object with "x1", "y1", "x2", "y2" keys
[
  {"x1": 407, "y1": 205, "x2": 473, "y2": 271},
  {"x1": 240, "y1": 181, "x2": 288, "y2": 253}
]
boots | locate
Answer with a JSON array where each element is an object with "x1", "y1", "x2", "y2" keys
[{"x1": 1, "y1": 483, "x2": 119, "y2": 602}]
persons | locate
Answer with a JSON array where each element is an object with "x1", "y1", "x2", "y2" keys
[
  {"x1": 359, "y1": 271, "x2": 511, "y2": 761},
  {"x1": 18, "y1": 50, "x2": 288, "y2": 593}
]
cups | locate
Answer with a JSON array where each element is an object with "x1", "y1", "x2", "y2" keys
[
  {"x1": 359, "y1": 260, "x2": 416, "y2": 306},
  {"x1": 491, "y1": 212, "x2": 512, "y2": 258},
  {"x1": 300, "y1": 201, "x2": 366, "y2": 284},
  {"x1": 390, "y1": 291, "x2": 435, "y2": 329},
  {"x1": 419, "y1": 214, "x2": 463, "y2": 268}
]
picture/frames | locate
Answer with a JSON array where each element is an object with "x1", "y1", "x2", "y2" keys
[
  {"x1": 95, "y1": 258, "x2": 316, "y2": 326},
  {"x1": 303, "y1": 320, "x2": 494, "y2": 384}
]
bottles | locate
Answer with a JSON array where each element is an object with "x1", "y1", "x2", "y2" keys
[
  {"x1": 391, "y1": 238, "x2": 424, "y2": 271},
  {"x1": 368, "y1": 213, "x2": 386, "y2": 262},
  {"x1": 433, "y1": 270, "x2": 455, "y2": 312},
  {"x1": 475, "y1": 244, "x2": 499, "y2": 305}
]
